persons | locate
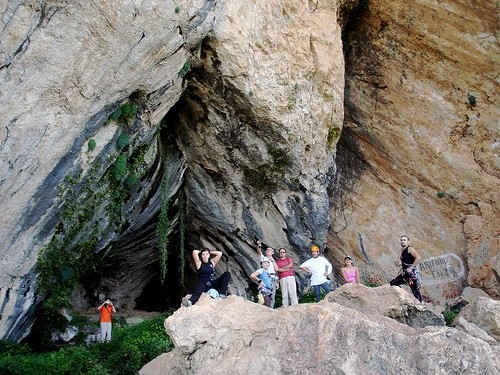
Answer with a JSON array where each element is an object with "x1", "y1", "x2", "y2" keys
[
  {"x1": 273, "y1": 248, "x2": 298, "y2": 307},
  {"x1": 389, "y1": 234, "x2": 423, "y2": 304},
  {"x1": 257, "y1": 240, "x2": 278, "y2": 309},
  {"x1": 97, "y1": 298, "x2": 116, "y2": 343},
  {"x1": 250, "y1": 256, "x2": 273, "y2": 308},
  {"x1": 341, "y1": 255, "x2": 360, "y2": 284},
  {"x1": 298, "y1": 245, "x2": 333, "y2": 303},
  {"x1": 182, "y1": 247, "x2": 231, "y2": 307}
]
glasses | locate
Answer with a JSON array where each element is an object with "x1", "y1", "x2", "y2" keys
[{"x1": 281, "y1": 252, "x2": 286, "y2": 253}]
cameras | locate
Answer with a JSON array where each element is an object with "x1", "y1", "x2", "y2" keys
[
  {"x1": 106, "y1": 301, "x2": 109, "y2": 304},
  {"x1": 258, "y1": 244, "x2": 261, "y2": 248},
  {"x1": 327, "y1": 277, "x2": 331, "y2": 280}
]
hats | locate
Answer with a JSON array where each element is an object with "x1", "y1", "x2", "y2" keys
[
  {"x1": 260, "y1": 257, "x2": 271, "y2": 264},
  {"x1": 208, "y1": 289, "x2": 219, "y2": 299},
  {"x1": 345, "y1": 256, "x2": 352, "y2": 260},
  {"x1": 104, "y1": 299, "x2": 111, "y2": 304}
]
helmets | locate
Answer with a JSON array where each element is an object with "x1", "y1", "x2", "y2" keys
[{"x1": 311, "y1": 246, "x2": 319, "y2": 252}]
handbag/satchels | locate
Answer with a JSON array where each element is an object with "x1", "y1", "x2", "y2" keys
[{"x1": 257, "y1": 290, "x2": 264, "y2": 305}]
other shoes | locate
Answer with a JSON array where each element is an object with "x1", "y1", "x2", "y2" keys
[{"x1": 182, "y1": 298, "x2": 192, "y2": 307}]
